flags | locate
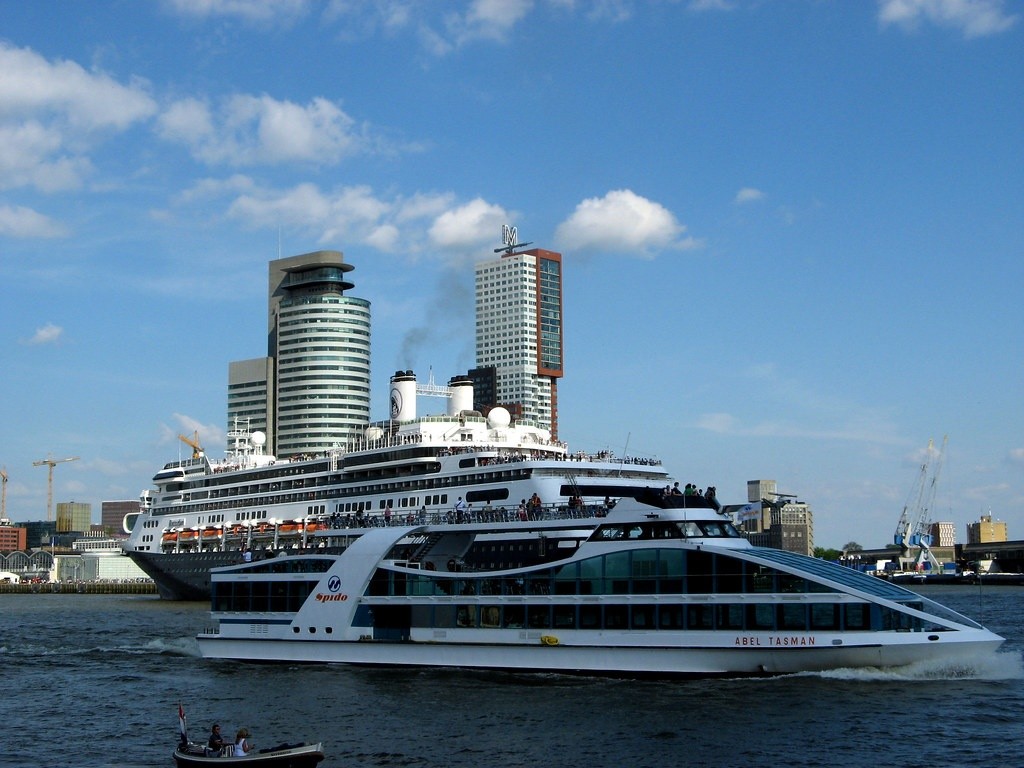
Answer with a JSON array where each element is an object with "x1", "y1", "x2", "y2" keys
[{"x1": 732, "y1": 501, "x2": 762, "y2": 526}]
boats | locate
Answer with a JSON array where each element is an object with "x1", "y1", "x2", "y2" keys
[
  {"x1": 202, "y1": 529, "x2": 221, "y2": 540},
  {"x1": 306, "y1": 523, "x2": 327, "y2": 536},
  {"x1": 172, "y1": 700, "x2": 326, "y2": 768},
  {"x1": 162, "y1": 533, "x2": 177, "y2": 544},
  {"x1": 278, "y1": 524, "x2": 303, "y2": 536},
  {"x1": 180, "y1": 531, "x2": 199, "y2": 544},
  {"x1": 187, "y1": 490, "x2": 1006, "y2": 678}
]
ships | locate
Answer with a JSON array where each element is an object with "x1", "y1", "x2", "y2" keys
[{"x1": 112, "y1": 411, "x2": 728, "y2": 598}]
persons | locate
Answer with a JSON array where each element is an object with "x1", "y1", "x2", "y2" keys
[
  {"x1": 332, "y1": 506, "x2": 377, "y2": 528},
  {"x1": 208, "y1": 724, "x2": 224, "y2": 758},
  {"x1": 279, "y1": 544, "x2": 321, "y2": 558},
  {"x1": 663, "y1": 482, "x2": 717, "y2": 499},
  {"x1": 407, "y1": 513, "x2": 415, "y2": 526},
  {"x1": 213, "y1": 450, "x2": 330, "y2": 475},
  {"x1": 419, "y1": 505, "x2": 426, "y2": 525},
  {"x1": 233, "y1": 728, "x2": 255, "y2": 757},
  {"x1": 382, "y1": 505, "x2": 391, "y2": 527},
  {"x1": 243, "y1": 548, "x2": 253, "y2": 562},
  {"x1": 568, "y1": 494, "x2": 616, "y2": 517},
  {"x1": 439, "y1": 443, "x2": 662, "y2": 466},
  {"x1": 448, "y1": 493, "x2": 543, "y2": 525}
]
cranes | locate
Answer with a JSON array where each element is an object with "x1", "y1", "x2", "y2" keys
[
  {"x1": 0, "y1": 467, "x2": 9, "y2": 521},
  {"x1": 894, "y1": 437, "x2": 935, "y2": 547},
  {"x1": 32, "y1": 453, "x2": 85, "y2": 521},
  {"x1": 909, "y1": 435, "x2": 951, "y2": 548}
]
